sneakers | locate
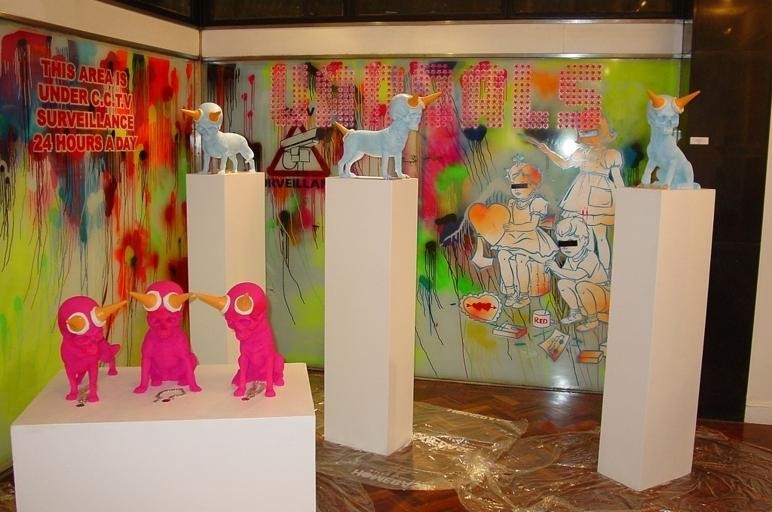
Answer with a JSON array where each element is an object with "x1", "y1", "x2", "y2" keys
[
  {"x1": 560, "y1": 314, "x2": 582, "y2": 324},
  {"x1": 505, "y1": 293, "x2": 530, "y2": 309},
  {"x1": 576, "y1": 319, "x2": 599, "y2": 331}
]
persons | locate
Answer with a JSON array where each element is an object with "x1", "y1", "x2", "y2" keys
[
  {"x1": 536, "y1": 111, "x2": 627, "y2": 277},
  {"x1": 488, "y1": 163, "x2": 559, "y2": 310},
  {"x1": 544, "y1": 216, "x2": 612, "y2": 334}
]
